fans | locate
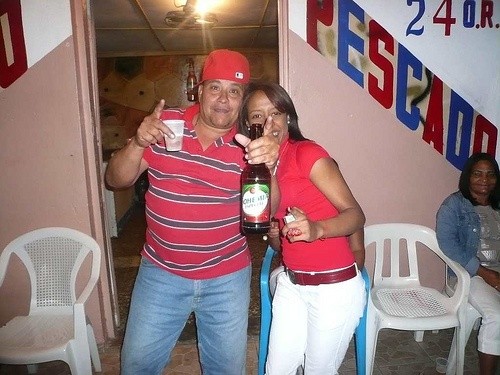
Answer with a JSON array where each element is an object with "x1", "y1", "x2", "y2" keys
[{"x1": 164, "y1": 0, "x2": 219, "y2": 30}]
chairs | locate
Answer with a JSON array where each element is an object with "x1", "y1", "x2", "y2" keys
[
  {"x1": 0, "y1": 227, "x2": 101, "y2": 374},
  {"x1": 261, "y1": 222, "x2": 483, "y2": 375}
]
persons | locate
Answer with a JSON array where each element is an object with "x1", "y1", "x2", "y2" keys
[
  {"x1": 105, "y1": 48, "x2": 281, "y2": 375},
  {"x1": 238, "y1": 82, "x2": 367, "y2": 375},
  {"x1": 436, "y1": 152, "x2": 500, "y2": 375}
]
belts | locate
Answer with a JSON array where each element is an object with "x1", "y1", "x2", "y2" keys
[{"x1": 284, "y1": 265, "x2": 358, "y2": 285}]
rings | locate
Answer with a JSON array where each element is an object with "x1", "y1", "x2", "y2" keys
[
  {"x1": 287, "y1": 227, "x2": 302, "y2": 237},
  {"x1": 495, "y1": 286, "x2": 498, "y2": 289}
]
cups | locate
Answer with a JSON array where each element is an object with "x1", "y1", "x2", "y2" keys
[{"x1": 162, "y1": 120, "x2": 185, "y2": 152}]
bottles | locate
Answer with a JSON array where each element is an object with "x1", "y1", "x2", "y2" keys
[
  {"x1": 241, "y1": 124, "x2": 271, "y2": 235},
  {"x1": 187, "y1": 60, "x2": 198, "y2": 101}
]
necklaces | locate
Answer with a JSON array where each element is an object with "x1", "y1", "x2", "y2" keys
[{"x1": 273, "y1": 141, "x2": 289, "y2": 177}]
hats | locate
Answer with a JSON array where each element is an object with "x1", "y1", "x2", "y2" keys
[{"x1": 193, "y1": 49, "x2": 251, "y2": 85}]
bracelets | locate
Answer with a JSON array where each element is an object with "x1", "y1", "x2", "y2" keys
[{"x1": 317, "y1": 220, "x2": 327, "y2": 241}]
